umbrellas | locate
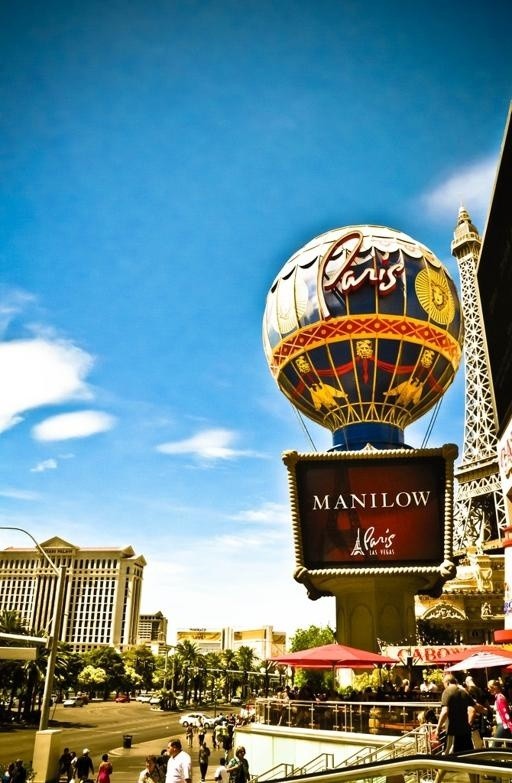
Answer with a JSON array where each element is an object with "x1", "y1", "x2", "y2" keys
[
  {"x1": 265, "y1": 640, "x2": 399, "y2": 688},
  {"x1": 441, "y1": 650, "x2": 512, "y2": 683}
]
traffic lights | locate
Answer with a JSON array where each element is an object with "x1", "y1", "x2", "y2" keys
[
  {"x1": 222, "y1": 669, "x2": 226, "y2": 676},
  {"x1": 134, "y1": 657, "x2": 140, "y2": 666},
  {"x1": 150, "y1": 663, "x2": 155, "y2": 671},
  {"x1": 244, "y1": 671, "x2": 246, "y2": 677},
  {"x1": 203, "y1": 669, "x2": 206, "y2": 676},
  {"x1": 144, "y1": 661, "x2": 148, "y2": 669}
]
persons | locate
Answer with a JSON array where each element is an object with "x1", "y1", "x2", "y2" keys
[
  {"x1": 499, "y1": 446, "x2": 511, "y2": 471},
  {"x1": 10, "y1": 758, "x2": 27, "y2": 782},
  {"x1": 54, "y1": 746, "x2": 113, "y2": 783},
  {"x1": 1, "y1": 762, "x2": 15, "y2": 783},
  {"x1": 271, "y1": 672, "x2": 439, "y2": 726},
  {"x1": 416, "y1": 673, "x2": 512, "y2": 780},
  {"x1": 156, "y1": 701, "x2": 256, "y2": 783},
  {"x1": 137, "y1": 754, "x2": 166, "y2": 783}
]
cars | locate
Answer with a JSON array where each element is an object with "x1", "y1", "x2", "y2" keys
[
  {"x1": 230, "y1": 695, "x2": 241, "y2": 703},
  {"x1": 135, "y1": 690, "x2": 212, "y2": 705},
  {"x1": 116, "y1": 695, "x2": 130, "y2": 702},
  {"x1": 178, "y1": 712, "x2": 216, "y2": 729},
  {"x1": 63, "y1": 696, "x2": 86, "y2": 707},
  {"x1": 0, "y1": 693, "x2": 60, "y2": 709}
]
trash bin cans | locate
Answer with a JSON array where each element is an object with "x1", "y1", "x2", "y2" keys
[{"x1": 123, "y1": 734, "x2": 132, "y2": 748}]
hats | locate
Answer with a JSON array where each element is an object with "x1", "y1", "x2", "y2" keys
[
  {"x1": 82, "y1": 747, "x2": 90, "y2": 754},
  {"x1": 15, "y1": 758, "x2": 24, "y2": 763}
]
